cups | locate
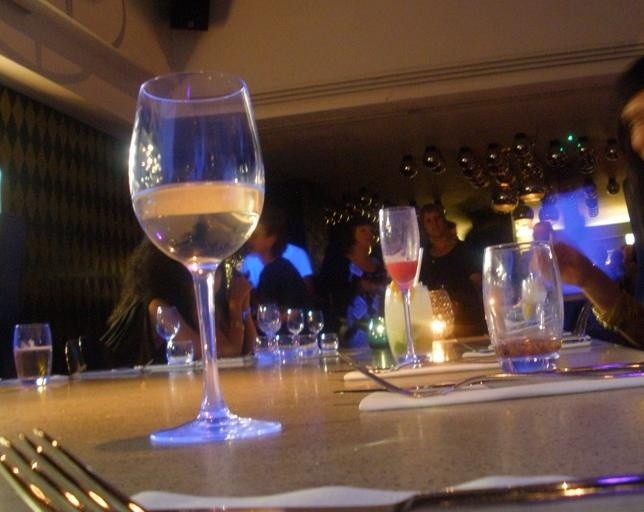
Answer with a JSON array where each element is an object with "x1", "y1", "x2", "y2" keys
[
  {"x1": 14, "y1": 321, "x2": 54, "y2": 390},
  {"x1": 384, "y1": 284, "x2": 433, "y2": 368},
  {"x1": 485, "y1": 242, "x2": 565, "y2": 374},
  {"x1": 320, "y1": 333, "x2": 339, "y2": 359}
]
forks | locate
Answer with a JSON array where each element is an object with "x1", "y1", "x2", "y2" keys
[
  {"x1": 9, "y1": 432, "x2": 644, "y2": 512},
  {"x1": 339, "y1": 349, "x2": 644, "y2": 398}
]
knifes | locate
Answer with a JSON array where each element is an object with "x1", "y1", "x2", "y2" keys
[{"x1": 333, "y1": 362, "x2": 644, "y2": 396}]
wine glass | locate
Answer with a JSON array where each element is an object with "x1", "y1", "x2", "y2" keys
[
  {"x1": 130, "y1": 72, "x2": 282, "y2": 443},
  {"x1": 377, "y1": 208, "x2": 424, "y2": 368},
  {"x1": 254, "y1": 302, "x2": 325, "y2": 357}
]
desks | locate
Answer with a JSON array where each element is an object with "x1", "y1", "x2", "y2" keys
[{"x1": 0, "y1": 329, "x2": 644, "y2": 512}]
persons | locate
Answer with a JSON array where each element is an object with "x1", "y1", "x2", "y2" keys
[
  {"x1": 534, "y1": 56, "x2": 644, "y2": 349},
  {"x1": 100, "y1": 194, "x2": 644, "y2": 366}
]
